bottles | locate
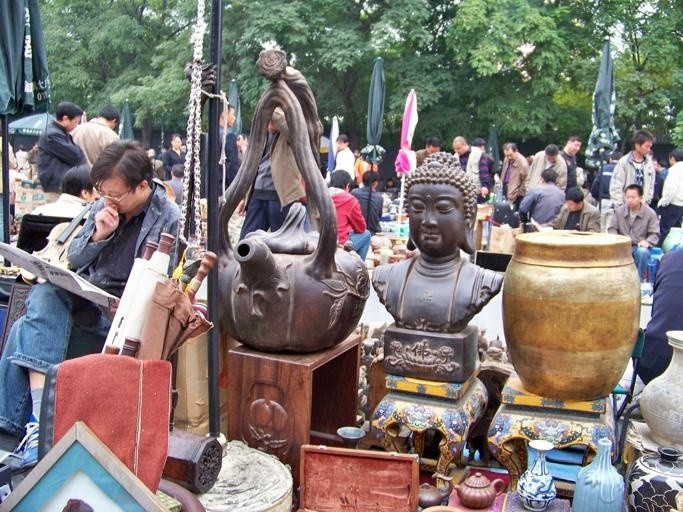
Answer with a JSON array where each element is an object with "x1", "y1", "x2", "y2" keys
[
  {"x1": 367, "y1": 231, "x2": 407, "y2": 266},
  {"x1": 336, "y1": 425, "x2": 365, "y2": 451},
  {"x1": 513, "y1": 435, "x2": 683, "y2": 512}
]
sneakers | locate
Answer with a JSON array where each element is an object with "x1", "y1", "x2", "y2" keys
[{"x1": 21, "y1": 411, "x2": 52, "y2": 468}]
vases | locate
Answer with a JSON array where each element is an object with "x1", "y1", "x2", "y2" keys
[
  {"x1": 516, "y1": 440, "x2": 559, "y2": 512},
  {"x1": 340, "y1": 427, "x2": 366, "y2": 451},
  {"x1": 572, "y1": 438, "x2": 624, "y2": 512},
  {"x1": 625, "y1": 447, "x2": 683, "y2": 512}
]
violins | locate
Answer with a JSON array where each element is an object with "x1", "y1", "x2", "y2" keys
[{"x1": 21, "y1": 202, "x2": 94, "y2": 285}]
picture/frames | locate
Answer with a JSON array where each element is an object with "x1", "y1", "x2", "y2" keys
[{"x1": 0, "y1": 421, "x2": 173, "y2": 512}]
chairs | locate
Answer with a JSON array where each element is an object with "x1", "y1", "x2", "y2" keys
[
  {"x1": 39, "y1": 354, "x2": 207, "y2": 512},
  {"x1": 610, "y1": 327, "x2": 645, "y2": 464},
  {"x1": 15, "y1": 214, "x2": 87, "y2": 254}
]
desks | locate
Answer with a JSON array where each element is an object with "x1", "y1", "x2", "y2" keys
[{"x1": 474, "y1": 203, "x2": 495, "y2": 251}]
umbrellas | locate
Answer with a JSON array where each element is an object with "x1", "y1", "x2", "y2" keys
[
  {"x1": 227, "y1": 77, "x2": 243, "y2": 135},
  {"x1": 584, "y1": 35, "x2": 622, "y2": 170},
  {"x1": 359, "y1": 56, "x2": 388, "y2": 165},
  {"x1": 0, "y1": 0, "x2": 53, "y2": 266}
]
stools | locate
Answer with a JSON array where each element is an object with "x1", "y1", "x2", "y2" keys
[
  {"x1": 371, "y1": 365, "x2": 489, "y2": 495},
  {"x1": 486, "y1": 370, "x2": 621, "y2": 492}
]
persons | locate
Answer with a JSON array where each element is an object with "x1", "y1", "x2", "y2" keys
[{"x1": 2, "y1": 100, "x2": 683, "y2": 474}]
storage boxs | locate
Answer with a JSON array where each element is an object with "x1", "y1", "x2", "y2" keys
[
  {"x1": 227, "y1": 334, "x2": 363, "y2": 504},
  {"x1": 489, "y1": 222, "x2": 524, "y2": 254},
  {"x1": 171, "y1": 299, "x2": 238, "y2": 442}
]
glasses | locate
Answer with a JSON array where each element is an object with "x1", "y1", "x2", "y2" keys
[{"x1": 92, "y1": 185, "x2": 135, "y2": 204}]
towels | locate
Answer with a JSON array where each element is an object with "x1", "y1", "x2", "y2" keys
[{"x1": 51, "y1": 353, "x2": 172, "y2": 495}]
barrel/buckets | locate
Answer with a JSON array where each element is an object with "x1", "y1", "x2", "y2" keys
[{"x1": 501, "y1": 229, "x2": 640, "y2": 401}]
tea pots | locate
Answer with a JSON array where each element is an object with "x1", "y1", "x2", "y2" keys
[
  {"x1": 218, "y1": 44, "x2": 371, "y2": 355},
  {"x1": 418, "y1": 472, "x2": 453, "y2": 508},
  {"x1": 453, "y1": 472, "x2": 505, "y2": 509}
]
